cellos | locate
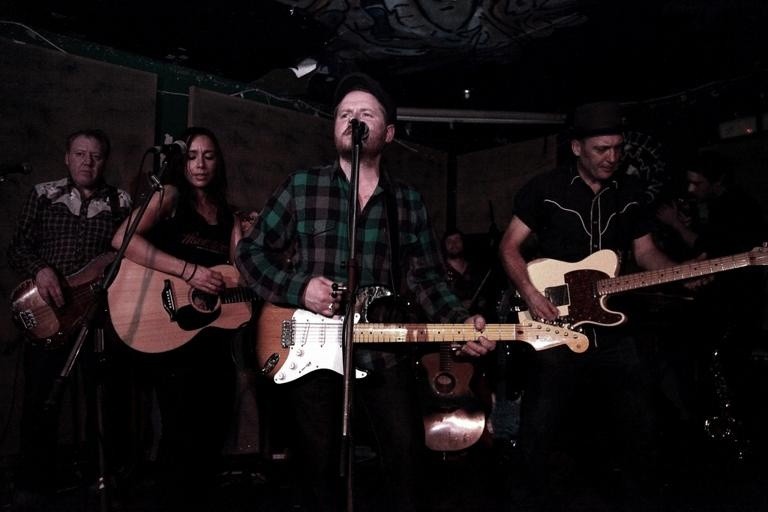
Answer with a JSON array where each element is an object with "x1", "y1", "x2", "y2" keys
[{"x1": 419, "y1": 263, "x2": 489, "y2": 452}]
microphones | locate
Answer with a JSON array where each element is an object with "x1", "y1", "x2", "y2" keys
[
  {"x1": 151, "y1": 140, "x2": 187, "y2": 154},
  {"x1": 352, "y1": 119, "x2": 369, "y2": 140}
]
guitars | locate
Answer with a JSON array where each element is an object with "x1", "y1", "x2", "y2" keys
[
  {"x1": 106, "y1": 256, "x2": 259, "y2": 354},
  {"x1": 253, "y1": 285, "x2": 590, "y2": 384},
  {"x1": 11, "y1": 209, "x2": 260, "y2": 340},
  {"x1": 508, "y1": 242, "x2": 766, "y2": 331}
]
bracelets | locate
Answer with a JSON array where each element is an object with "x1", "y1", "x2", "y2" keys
[
  {"x1": 180, "y1": 261, "x2": 187, "y2": 278},
  {"x1": 185, "y1": 264, "x2": 199, "y2": 282}
]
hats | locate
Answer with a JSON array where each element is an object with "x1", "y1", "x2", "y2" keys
[
  {"x1": 571, "y1": 99, "x2": 624, "y2": 138},
  {"x1": 332, "y1": 72, "x2": 397, "y2": 124}
]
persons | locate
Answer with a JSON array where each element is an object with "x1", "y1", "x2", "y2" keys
[
  {"x1": 7, "y1": 126, "x2": 140, "y2": 507},
  {"x1": 111, "y1": 127, "x2": 244, "y2": 512},
  {"x1": 441, "y1": 228, "x2": 487, "y2": 312},
  {"x1": 499, "y1": 101, "x2": 713, "y2": 512},
  {"x1": 655, "y1": 150, "x2": 764, "y2": 247},
  {"x1": 234, "y1": 73, "x2": 497, "y2": 512}
]
